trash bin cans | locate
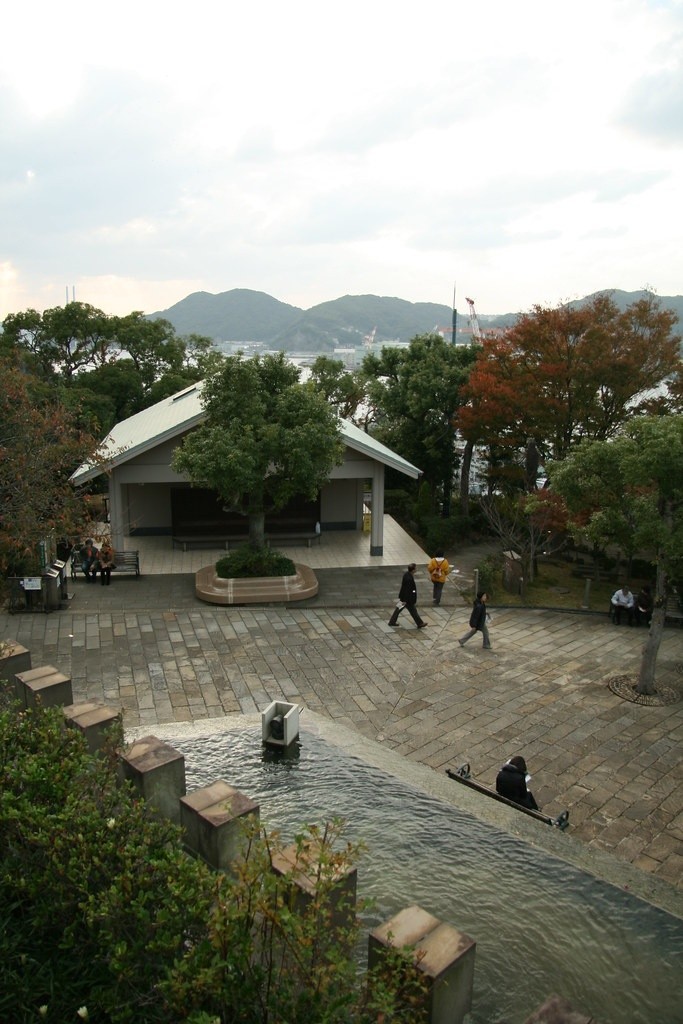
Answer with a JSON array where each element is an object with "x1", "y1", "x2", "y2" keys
[
  {"x1": 502, "y1": 551, "x2": 526, "y2": 594},
  {"x1": 42, "y1": 559, "x2": 76, "y2": 611}
]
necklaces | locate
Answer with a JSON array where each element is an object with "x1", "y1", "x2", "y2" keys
[{"x1": 495, "y1": 754, "x2": 540, "y2": 811}]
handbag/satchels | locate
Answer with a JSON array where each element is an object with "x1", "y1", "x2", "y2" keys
[{"x1": 111, "y1": 563, "x2": 117, "y2": 569}]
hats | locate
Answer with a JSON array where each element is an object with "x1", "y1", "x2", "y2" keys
[{"x1": 477, "y1": 590, "x2": 485, "y2": 599}]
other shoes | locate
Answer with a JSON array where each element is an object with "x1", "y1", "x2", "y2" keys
[
  {"x1": 482, "y1": 644, "x2": 492, "y2": 648},
  {"x1": 627, "y1": 621, "x2": 633, "y2": 626},
  {"x1": 418, "y1": 622, "x2": 428, "y2": 628},
  {"x1": 101, "y1": 576, "x2": 111, "y2": 585},
  {"x1": 614, "y1": 621, "x2": 620, "y2": 625},
  {"x1": 388, "y1": 621, "x2": 399, "y2": 626},
  {"x1": 458, "y1": 639, "x2": 463, "y2": 647},
  {"x1": 85, "y1": 575, "x2": 96, "y2": 583},
  {"x1": 433, "y1": 602, "x2": 439, "y2": 606}
]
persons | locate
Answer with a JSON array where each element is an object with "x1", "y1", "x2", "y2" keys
[
  {"x1": 386, "y1": 562, "x2": 429, "y2": 628},
  {"x1": 98, "y1": 542, "x2": 118, "y2": 586},
  {"x1": 458, "y1": 589, "x2": 494, "y2": 650},
  {"x1": 427, "y1": 548, "x2": 452, "y2": 604},
  {"x1": 79, "y1": 538, "x2": 99, "y2": 584},
  {"x1": 634, "y1": 585, "x2": 655, "y2": 627},
  {"x1": 610, "y1": 583, "x2": 636, "y2": 627}
]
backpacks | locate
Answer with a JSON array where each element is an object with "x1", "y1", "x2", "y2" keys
[{"x1": 431, "y1": 559, "x2": 445, "y2": 579}]
